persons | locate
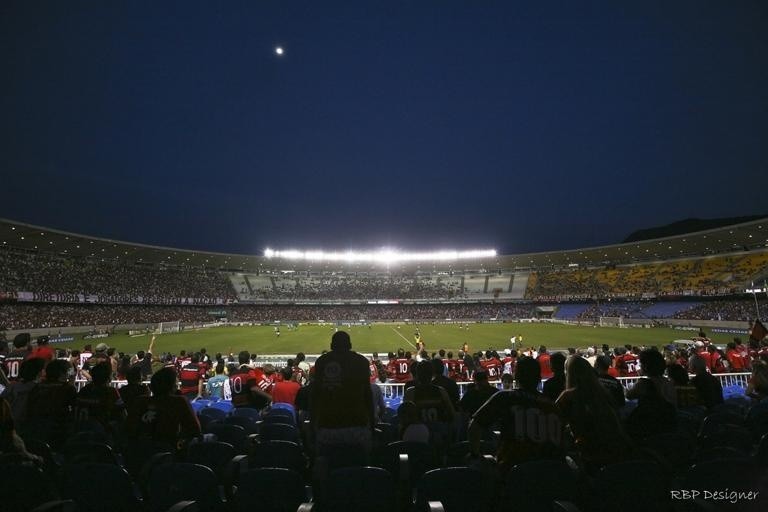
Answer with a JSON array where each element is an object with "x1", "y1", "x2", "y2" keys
[{"x1": 0, "y1": 318, "x2": 768, "y2": 512}]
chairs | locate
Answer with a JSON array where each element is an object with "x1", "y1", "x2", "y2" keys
[
  {"x1": 0, "y1": 349, "x2": 768, "y2": 509},
  {"x1": 221, "y1": 247, "x2": 768, "y2": 327}
]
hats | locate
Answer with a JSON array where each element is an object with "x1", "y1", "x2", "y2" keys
[{"x1": 95, "y1": 343, "x2": 111, "y2": 351}]
